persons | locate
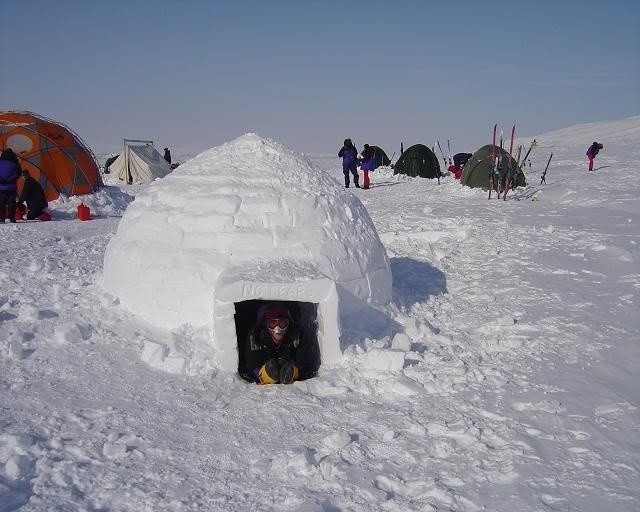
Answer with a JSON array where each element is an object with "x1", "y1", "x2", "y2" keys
[
  {"x1": 239, "y1": 302, "x2": 317, "y2": 384},
  {"x1": 338, "y1": 139, "x2": 377, "y2": 189},
  {"x1": 164, "y1": 148, "x2": 171, "y2": 164},
  {"x1": 0, "y1": 148, "x2": 48, "y2": 224},
  {"x1": 586, "y1": 142, "x2": 603, "y2": 171},
  {"x1": 453, "y1": 153, "x2": 473, "y2": 179}
]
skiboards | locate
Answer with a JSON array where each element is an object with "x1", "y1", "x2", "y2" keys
[
  {"x1": 381, "y1": 141, "x2": 405, "y2": 166},
  {"x1": 437, "y1": 122, "x2": 553, "y2": 200}
]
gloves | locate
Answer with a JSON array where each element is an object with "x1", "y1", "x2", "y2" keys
[
  {"x1": 279, "y1": 359, "x2": 299, "y2": 384},
  {"x1": 258, "y1": 358, "x2": 281, "y2": 384}
]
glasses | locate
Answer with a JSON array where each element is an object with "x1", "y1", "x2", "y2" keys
[{"x1": 267, "y1": 319, "x2": 289, "y2": 331}]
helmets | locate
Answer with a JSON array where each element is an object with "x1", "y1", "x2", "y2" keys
[{"x1": 264, "y1": 305, "x2": 291, "y2": 319}]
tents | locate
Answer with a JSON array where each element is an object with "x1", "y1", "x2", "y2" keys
[
  {"x1": 108, "y1": 139, "x2": 172, "y2": 185},
  {"x1": 461, "y1": 145, "x2": 526, "y2": 192},
  {"x1": 394, "y1": 144, "x2": 442, "y2": 179},
  {"x1": 361, "y1": 146, "x2": 391, "y2": 168},
  {"x1": 0, "y1": 111, "x2": 105, "y2": 202}
]
